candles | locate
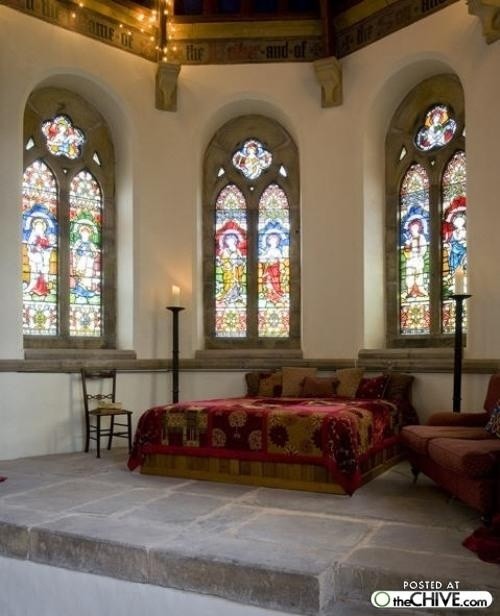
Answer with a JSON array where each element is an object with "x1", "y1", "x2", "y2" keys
[
  {"x1": 455, "y1": 265, "x2": 464, "y2": 295},
  {"x1": 171, "y1": 285, "x2": 180, "y2": 307}
]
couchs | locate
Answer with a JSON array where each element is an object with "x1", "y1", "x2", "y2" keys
[{"x1": 400, "y1": 373, "x2": 500, "y2": 520}]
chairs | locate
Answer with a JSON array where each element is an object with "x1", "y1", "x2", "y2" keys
[{"x1": 82, "y1": 368, "x2": 134, "y2": 458}]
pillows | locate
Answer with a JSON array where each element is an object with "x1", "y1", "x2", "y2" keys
[
  {"x1": 357, "y1": 372, "x2": 388, "y2": 400},
  {"x1": 246, "y1": 373, "x2": 281, "y2": 395},
  {"x1": 484, "y1": 371, "x2": 500, "y2": 415},
  {"x1": 302, "y1": 375, "x2": 337, "y2": 397},
  {"x1": 281, "y1": 367, "x2": 315, "y2": 397},
  {"x1": 382, "y1": 370, "x2": 413, "y2": 403},
  {"x1": 484, "y1": 399, "x2": 500, "y2": 438},
  {"x1": 334, "y1": 369, "x2": 362, "y2": 399}
]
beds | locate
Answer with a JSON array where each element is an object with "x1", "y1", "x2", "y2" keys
[{"x1": 139, "y1": 387, "x2": 417, "y2": 495}]
